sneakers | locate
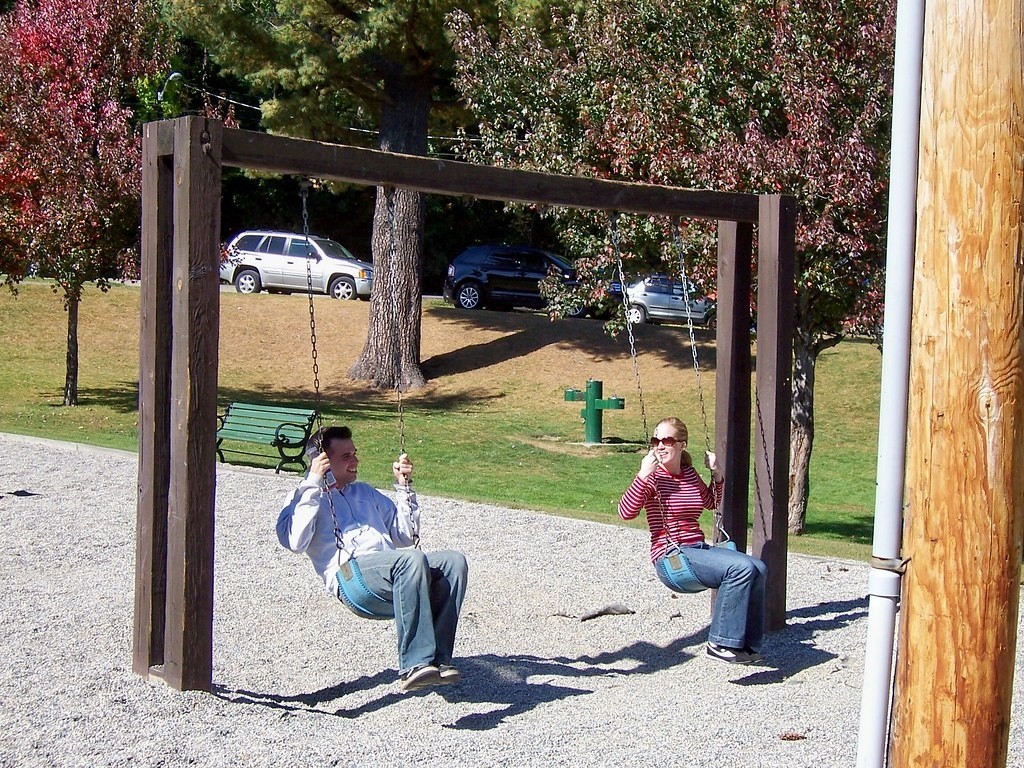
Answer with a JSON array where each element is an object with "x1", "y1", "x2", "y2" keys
[
  {"x1": 400, "y1": 665, "x2": 440, "y2": 692},
  {"x1": 432, "y1": 657, "x2": 460, "y2": 685},
  {"x1": 743, "y1": 643, "x2": 764, "y2": 664},
  {"x1": 706, "y1": 640, "x2": 752, "y2": 664}
]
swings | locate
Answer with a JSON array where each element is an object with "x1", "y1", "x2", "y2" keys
[
  {"x1": 604, "y1": 208, "x2": 739, "y2": 595},
  {"x1": 294, "y1": 174, "x2": 427, "y2": 622}
]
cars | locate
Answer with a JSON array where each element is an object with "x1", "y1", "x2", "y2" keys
[{"x1": 626, "y1": 273, "x2": 716, "y2": 332}]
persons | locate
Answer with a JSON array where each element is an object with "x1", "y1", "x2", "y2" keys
[
  {"x1": 275, "y1": 426, "x2": 469, "y2": 691},
  {"x1": 617, "y1": 417, "x2": 768, "y2": 664}
]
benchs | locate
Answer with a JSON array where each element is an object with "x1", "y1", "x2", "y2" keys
[{"x1": 217, "y1": 402, "x2": 316, "y2": 475}]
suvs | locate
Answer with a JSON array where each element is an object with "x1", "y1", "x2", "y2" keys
[
  {"x1": 219, "y1": 229, "x2": 374, "y2": 301},
  {"x1": 443, "y1": 243, "x2": 623, "y2": 322}
]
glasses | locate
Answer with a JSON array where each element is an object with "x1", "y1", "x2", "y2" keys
[{"x1": 650, "y1": 437, "x2": 682, "y2": 446}]
